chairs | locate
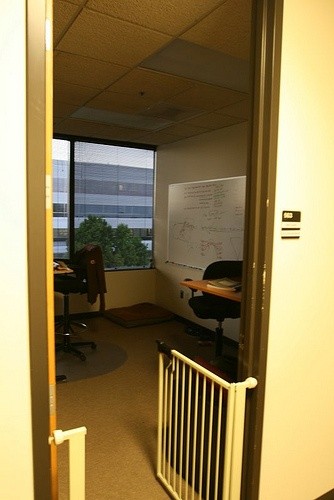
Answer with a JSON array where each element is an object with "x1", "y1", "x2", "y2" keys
[
  {"x1": 54, "y1": 244, "x2": 101, "y2": 362},
  {"x1": 184, "y1": 261, "x2": 244, "y2": 367}
]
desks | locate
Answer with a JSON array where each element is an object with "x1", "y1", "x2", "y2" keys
[{"x1": 180, "y1": 278, "x2": 242, "y2": 303}]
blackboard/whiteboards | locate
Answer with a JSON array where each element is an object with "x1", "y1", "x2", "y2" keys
[{"x1": 165, "y1": 175, "x2": 246, "y2": 271}]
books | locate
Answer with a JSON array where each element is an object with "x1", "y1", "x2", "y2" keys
[
  {"x1": 54, "y1": 267, "x2": 73, "y2": 274},
  {"x1": 207, "y1": 277, "x2": 241, "y2": 291}
]
